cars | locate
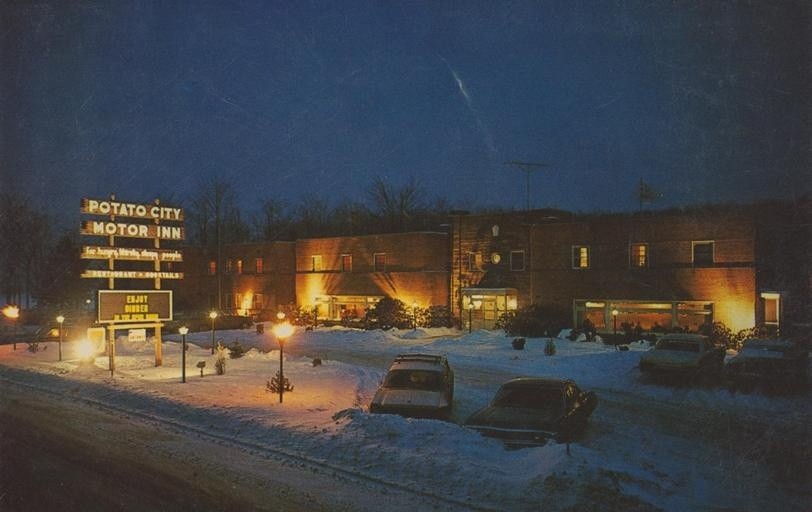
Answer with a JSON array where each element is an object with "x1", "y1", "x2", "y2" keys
[
  {"x1": 34, "y1": 324, "x2": 84, "y2": 342},
  {"x1": 461, "y1": 376, "x2": 601, "y2": 450},
  {"x1": 723, "y1": 335, "x2": 809, "y2": 395},
  {"x1": 637, "y1": 332, "x2": 730, "y2": 383},
  {"x1": 368, "y1": 349, "x2": 455, "y2": 421}
]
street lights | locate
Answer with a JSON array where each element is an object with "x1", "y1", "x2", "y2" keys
[
  {"x1": 208, "y1": 310, "x2": 219, "y2": 354},
  {"x1": 412, "y1": 300, "x2": 418, "y2": 331},
  {"x1": 55, "y1": 314, "x2": 65, "y2": 361},
  {"x1": 1, "y1": 303, "x2": 19, "y2": 351},
  {"x1": 467, "y1": 298, "x2": 475, "y2": 332},
  {"x1": 177, "y1": 324, "x2": 189, "y2": 384},
  {"x1": 612, "y1": 308, "x2": 618, "y2": 351},
  {"x1": 270, "y1": 321, "x2": 298, "y2": 403}
]
